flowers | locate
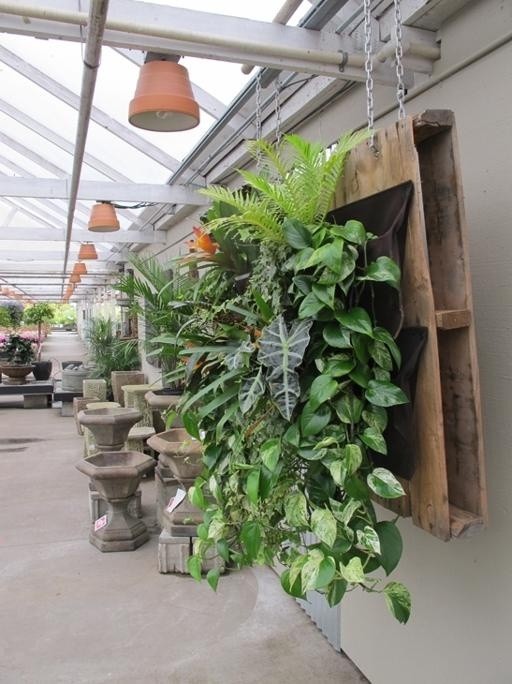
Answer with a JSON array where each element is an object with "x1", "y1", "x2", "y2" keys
[{"x1": 0, "y1": 331, "x2": 45, "y2": 365}]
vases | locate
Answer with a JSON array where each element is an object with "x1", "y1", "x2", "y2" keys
[
  {"x1": 73, "y1": 371, "x2": 227, "y2": 576},
  {"x1": 32, "y1": 361, "x2": 52, "y2": 380},
  {"x1": 0, "y1": 364, "x2": 37, "y2": 385}
]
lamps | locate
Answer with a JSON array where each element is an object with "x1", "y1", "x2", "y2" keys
[{"x1": 59, "y1": 56, "x2": 200, "y2": 305}]
